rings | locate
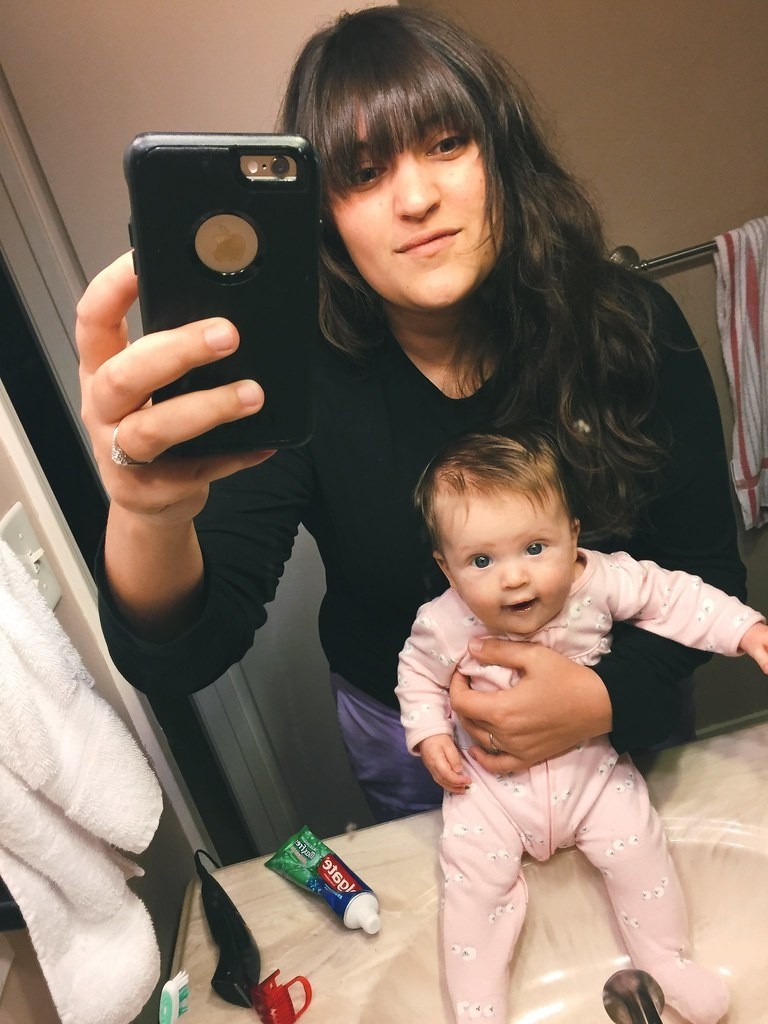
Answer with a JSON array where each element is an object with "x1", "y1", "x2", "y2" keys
[
  {"x1": 113, "y1": 420, "x2": 157, "y2": 468},
  {"x1": 489, "y1": 733, "x2": 500, "y2": 752}
]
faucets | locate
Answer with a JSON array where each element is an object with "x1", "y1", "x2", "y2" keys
[{"x1": 601, "y1": 968, "x2": 668, "y2": 1024}]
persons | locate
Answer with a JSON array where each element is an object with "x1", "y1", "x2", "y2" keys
[
  {"x1": 392, "y1": 431, "x2": 767, "y2": 1023},
  {"x1": 70, "y1": 3, "x2": 751, "y2": 822}
]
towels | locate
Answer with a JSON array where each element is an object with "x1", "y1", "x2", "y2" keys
[
  {"x1": 1, "y1": 536, "x2": 168, "y2": 1024},
  {"x1": 713, "y1": 211, "x2": 767, "y2": 529}
]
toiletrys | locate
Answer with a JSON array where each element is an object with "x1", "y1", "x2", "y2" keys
[{"x1": 261, "y1": 822, "x2": 385, "y2": 938}]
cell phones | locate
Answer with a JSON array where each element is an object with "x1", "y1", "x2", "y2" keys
[{"x1": 125, "y1": 132, "x2": 325, "y2": 457}]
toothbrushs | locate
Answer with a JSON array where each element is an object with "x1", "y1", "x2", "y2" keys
[{"x1": 157, "y1": 968, "x2": 191, "y2": 1024}]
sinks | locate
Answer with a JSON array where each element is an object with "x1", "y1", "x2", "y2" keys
[{"x1": 356, "y1": 817, "x2": 768, "y2": 1024}]
